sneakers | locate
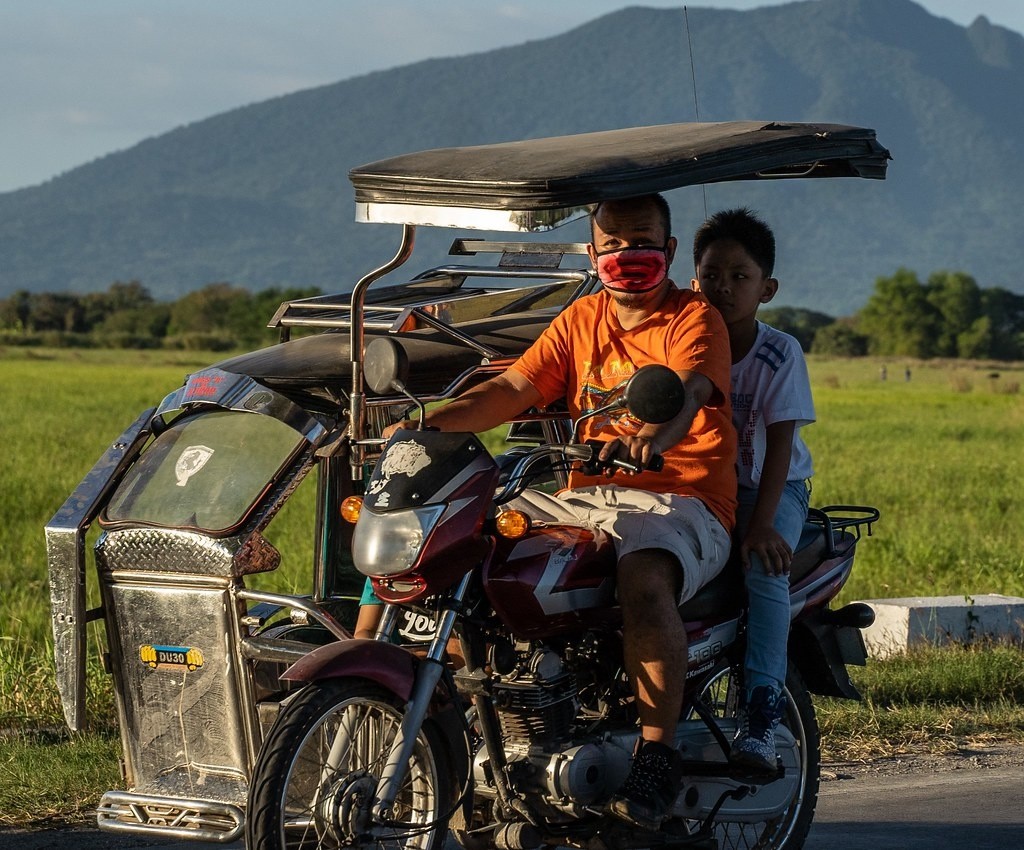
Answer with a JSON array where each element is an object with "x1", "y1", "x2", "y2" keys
[
  {"x1": 609, "y1": 740, "x2": 681, "y2": 831},
  {"x1": 730, "y1": 687, "x2": 787, "y2": 772}
]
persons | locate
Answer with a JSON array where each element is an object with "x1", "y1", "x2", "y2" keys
[
  {"x1": 688, "y1": 208, "x2": 817, "y2": 788},
  {"x1": 351, "y1": 575, "x2": 497, "y2": 676},
  {"x1": 377, "y1": 190, "x2": 737, "y2": 832}
]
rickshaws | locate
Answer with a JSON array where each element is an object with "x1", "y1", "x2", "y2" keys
[{"x1": 46, "y1": 119, "x2": 897, "y2": 849}]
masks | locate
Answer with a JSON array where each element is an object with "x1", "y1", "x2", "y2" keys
[{"x1": 592, "y1": 248, "x2": 667, "y2": 292}]
